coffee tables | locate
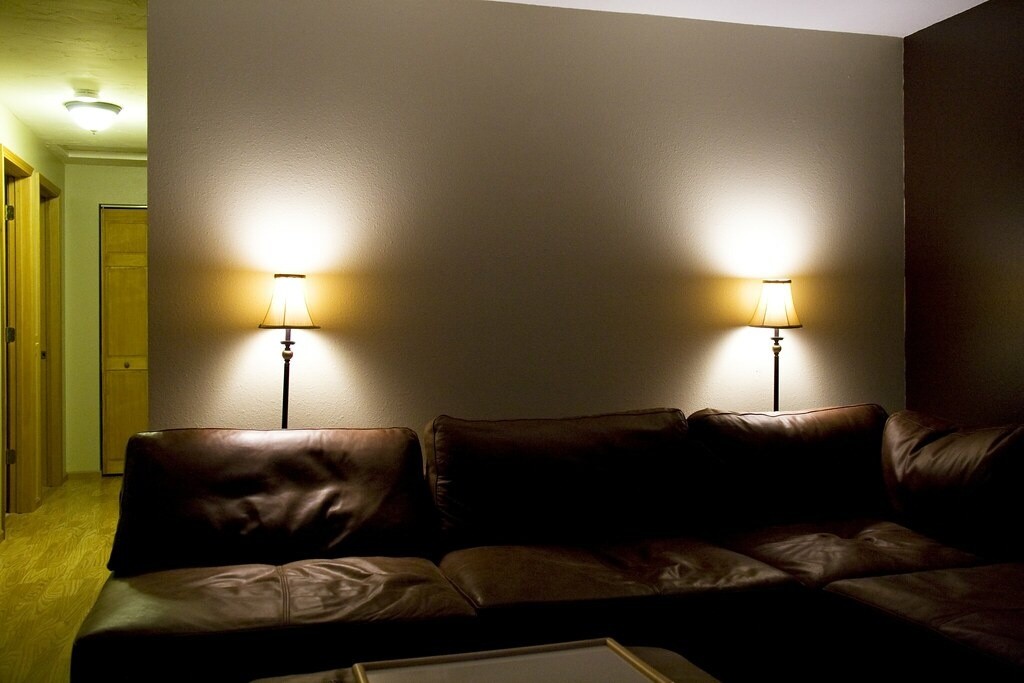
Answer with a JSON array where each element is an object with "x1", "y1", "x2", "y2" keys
[{"x1": 250, "y1": 647, "x2": 720, "y2": 683}]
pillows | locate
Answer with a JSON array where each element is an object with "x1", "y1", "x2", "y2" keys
[
  {"x1": 881, "y1": 409, "x2": 1024, "y2": 548},
  {"x1": 423, "y1": 407, "x2": 688, "y2": 546},
  {"x1": 686, "y1": 403, "x2": 888, "y2": 539},
  {"x1": 107, "y1": 427, "x2": 426, "y2": 575}
]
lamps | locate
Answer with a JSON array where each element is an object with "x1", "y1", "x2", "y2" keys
[
  {"x1": 258, "y1": 272, "x2": 322, "y2": 429},
  {"x1": 746, "y1": 278, "x2": 803, "y2": 412}
]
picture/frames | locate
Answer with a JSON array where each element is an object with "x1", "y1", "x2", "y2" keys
[{"x1": 351, "y1": 637, "x2": 673, "y2": 683}]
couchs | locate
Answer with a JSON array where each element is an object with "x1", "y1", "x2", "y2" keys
[
  {"x1": 815, "y1": 562, "x2": 1024, "y2": 683},
  {"x1": 68, "y1": 404, "x2": 1024, "y2": 683}
]
